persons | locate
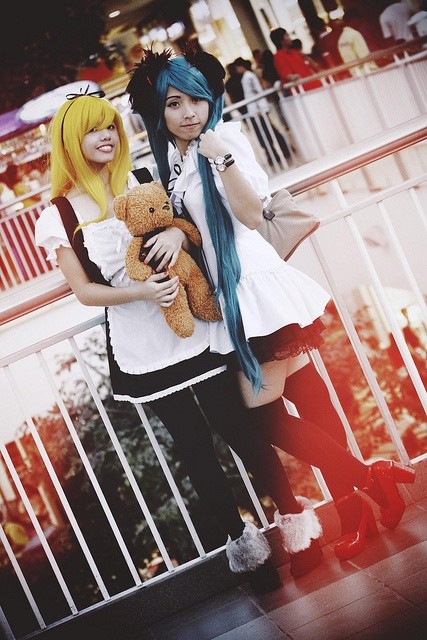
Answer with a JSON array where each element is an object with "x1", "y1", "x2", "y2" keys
[
  {"x1": 379, "y1": 0, "x2": 418, "y2": 43},
  {"x1": 130, "y1": 47, "x2": 416, "y2": 561},
  {"x1": 250, "y1": 48, "x2": 289, "y2": 131},
  {"x1": 269, "y1": 27, "x2": 324, "y2": 91},
  {"x1": 233, "y1": 56, "x2": 295, "y2": 168},
  {"x1": 292, "y1": 38, "x2": 323, "y2": 72},
  {"x1": 225, "y1": 62, "x2": 274, "y2": 166},
  {"x1": 310, "y1": 17, "x2": 350, "y2": 81},
  {"x1": 34, "y1": 86, "x2": 325, "y2": 598},
  {"x1": 261, "y1": 48, "x2": 292, "y2": 101},
  {"x1": 405, "y1": 0, "x2": 427, "y2": 42},
  {"x1": 330, "y1": 14, "x2": 380, "y2": 77}
]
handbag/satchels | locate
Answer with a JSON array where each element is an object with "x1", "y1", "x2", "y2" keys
[{"x1": 255, "y1": 189, "x2": 320, "y2": 262}]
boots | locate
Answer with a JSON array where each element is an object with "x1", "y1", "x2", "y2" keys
[
  {"x1": 225, "y1": 521, "x2": 280, "y2": 595},
  {"x1": 273, "y1": 496, "x2": 323, "y2": 577}
]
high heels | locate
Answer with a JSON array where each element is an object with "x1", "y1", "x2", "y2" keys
[
  {"x1": 364, "y1": 460, "x2": 416, "y2": 530},
  {"x1": 334, "y1": 491, "x2": 378, "y2": 559}
]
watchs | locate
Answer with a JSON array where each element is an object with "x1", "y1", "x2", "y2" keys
[
  {"x1": 216, "y1": 159, "x2": 236, "y2": 171},
  {"x1": 214, "y1": 153, "x2": 231, "y2": 165}
]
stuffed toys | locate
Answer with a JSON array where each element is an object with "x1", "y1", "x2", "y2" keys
[{"x1": 113, "y1": 180, "x2": 223, "y2": 337}]
